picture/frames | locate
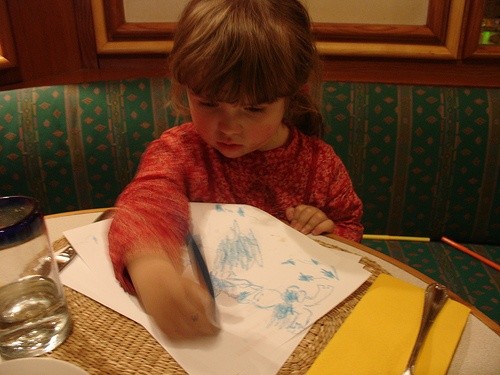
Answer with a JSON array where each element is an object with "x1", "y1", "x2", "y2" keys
[
  {"x1": 91, "y1": 0, "x2": 468, "y2": 62},
  {"x1": 0, "y1": 0, "x2": 21, "y2": 71},
  {"x1": 464, "y1": 0, "x2": 500, "y2": 61}
]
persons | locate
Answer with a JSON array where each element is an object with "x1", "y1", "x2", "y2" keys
[{"x1": 107, "y1": 0, "x2": 365, "y2": 345}]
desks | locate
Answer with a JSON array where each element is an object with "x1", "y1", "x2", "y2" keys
[{"x1": 0, "y1": 207, "x2": 499, "y2": 374}]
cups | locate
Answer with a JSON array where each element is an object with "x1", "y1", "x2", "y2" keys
[{"x1": 0, "y1": 195, "x2": 72, "y2": 360}]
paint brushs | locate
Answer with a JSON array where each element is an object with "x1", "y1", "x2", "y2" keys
[
  {"x1": 362, "y1": 235, "x2": 430, "y2": 241},
  {"x1": 183, "y1": 233, "x2": 216, "y2": 302},
  {"x1": 442, "y1": 237, "x2": 500, "y2": 269}
]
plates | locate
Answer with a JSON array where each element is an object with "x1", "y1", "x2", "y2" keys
[
  {"x1": 0, "y1": 195, "x2": 40, "y2": 245},
  {"x1": 0, "y1": 357, "x2": 90, "y2": 375}
]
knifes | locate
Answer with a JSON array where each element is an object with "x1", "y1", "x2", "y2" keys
[{"x1": 37, "y1": 209, "x2": 118, "y2": 278}]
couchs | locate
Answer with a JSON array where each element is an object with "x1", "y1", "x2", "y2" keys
[{"x1": 0, "y1": 80, "x2": 499, "y2": 330}]
